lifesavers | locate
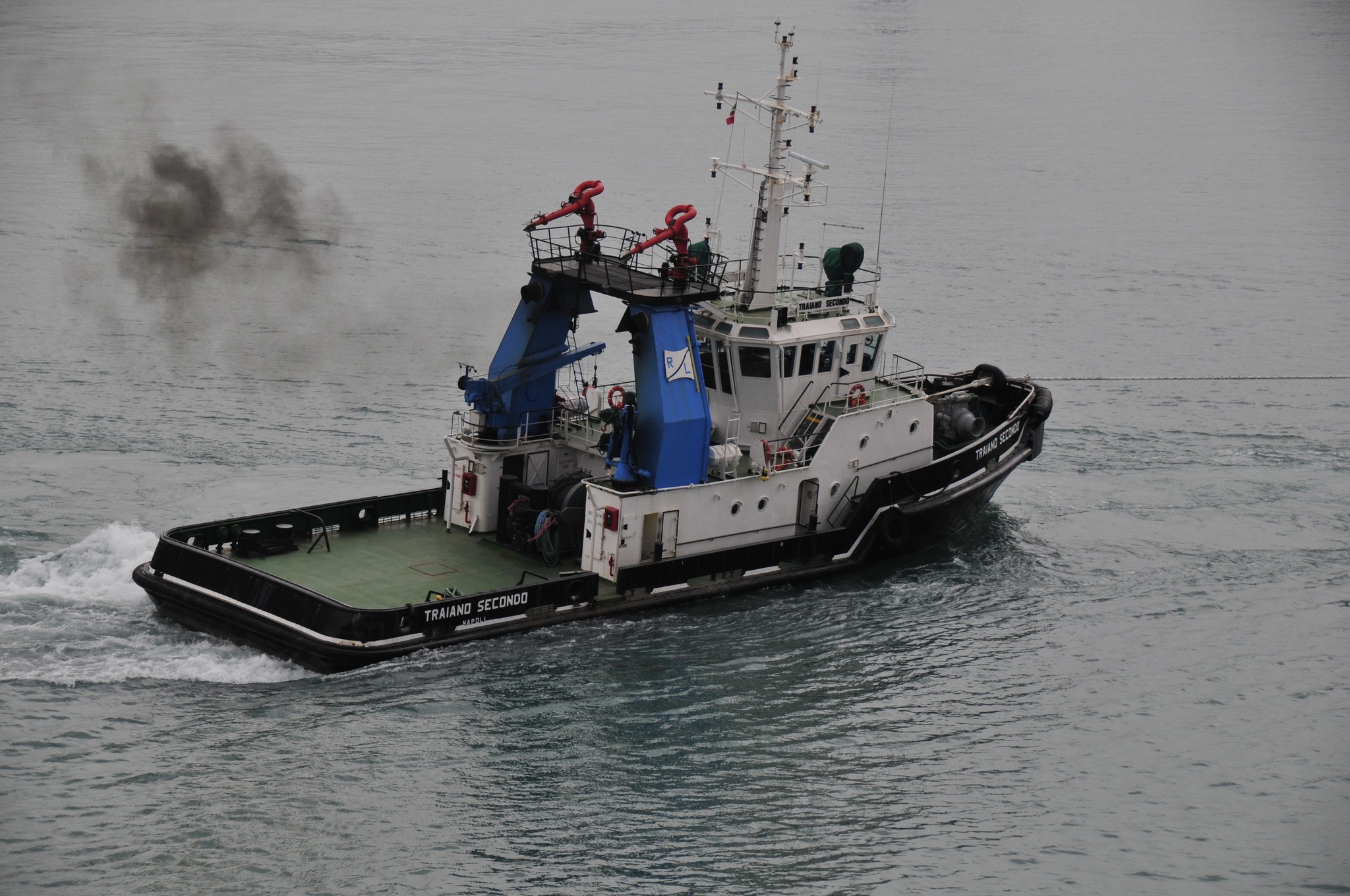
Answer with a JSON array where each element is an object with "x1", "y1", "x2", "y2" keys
[
  {"x1": 582, "y1": 385, "x2": 594, "y2": 397},
  {"x1": 757, "y1": 439, "x2": 771, "y2": 462},
  {"x1": 608, "y1": 385, "x2": 626, "y2": 409},
  {"x1": 772, "y1": 447, "x2": 792, "y2": 471},
  {"x1": 848, "y1": 384, "x2": 865, "y2": 408}
]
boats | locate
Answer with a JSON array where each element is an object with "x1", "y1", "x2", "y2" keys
[{"x1": 127, "y1": 13, "x2": 1054, "y2": 678}]
